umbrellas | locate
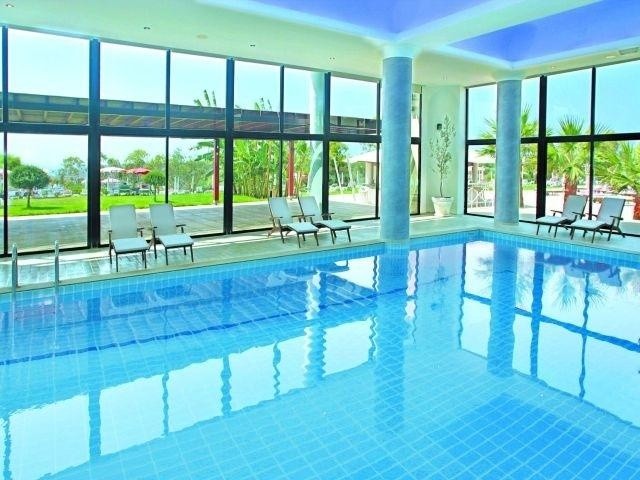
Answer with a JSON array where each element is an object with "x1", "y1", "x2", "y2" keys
[{"x1": 99, "y1": 166, "x2": 151, "y2": 192}]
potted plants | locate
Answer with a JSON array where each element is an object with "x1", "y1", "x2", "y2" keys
[{"x1": 427, "y1": 112, "x2": 458, "y2": 216}]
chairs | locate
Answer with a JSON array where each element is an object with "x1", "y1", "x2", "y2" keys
[
  {"x1": 535, "y1": 194, "x2": 626, "y2": 244},
  {"x1": 107, "y1": 203, "x2": 194, "y2": 272},
  {"x1": 537, "y1": 255, "x2": 622, "y2": 288},
  {"x1": 265, "y1": 268, "x2": 319, "y2": 288},
  {"x1": 268, "y1": 196, "x2": 352, "y2": 247}
]
